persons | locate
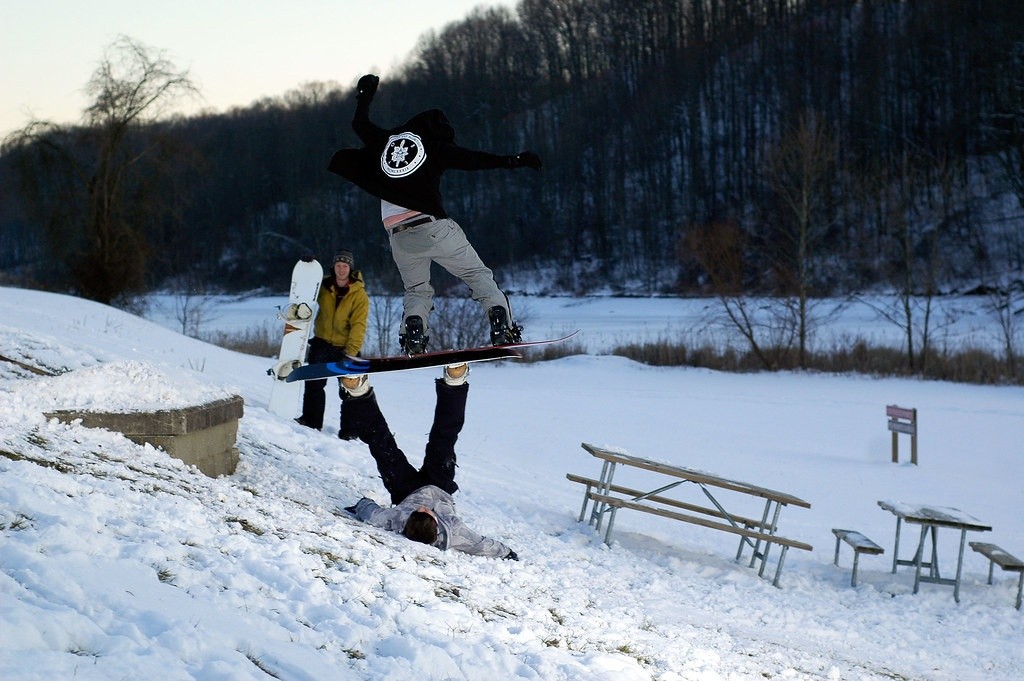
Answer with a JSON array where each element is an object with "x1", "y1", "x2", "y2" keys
[
  {"x1": 294, "y1": 253, "x2": 370, "y2": 440},
  {"x1": 326, "y1": 74, "x2": 531, "y2": 354},
  {"x1": 338, "y1": 363, "x2": 519, "y2": 561}
]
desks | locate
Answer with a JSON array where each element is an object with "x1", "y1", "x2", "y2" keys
[
  {"x1": 878, "y1": 500, "x2": 993, "y2": 603},
  {"x1": 581, "y1": 443, "x2": 811, "y2": 577}
]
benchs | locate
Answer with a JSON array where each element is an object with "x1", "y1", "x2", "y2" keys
[
  {"x1": 832, "y1": 529, "x2": 884, "y2": 587},
  {"x1": 969, "y1": 542, "x2": 1024, "y2": 609},
  {"x1": 567, "y1": 473, "x2": 777, "y2": 560},
  {"x1": 585, "y1": 492, "x2": 812, "y2": 587}
]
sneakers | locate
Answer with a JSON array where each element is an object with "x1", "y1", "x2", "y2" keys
[
  {"x1": 399, "y1": 315, "x2": 431, "y2": 358},
  {"x1": 444, "y1": 363, "x2": 470, "y2": 387},
  {"x1": 338, "y1": 374, "x2": 372, "y2": 400},
  {"x1": 489, "y1": 305, "x2": 523, "y2": 347}
]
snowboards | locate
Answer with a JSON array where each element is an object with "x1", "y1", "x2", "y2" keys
[
  {"x1": 287, "y1": 315, "x2": 585, "y2": 388},
  {"x1": 268, "y1": 253, "x2": 323, "y2": 418}
]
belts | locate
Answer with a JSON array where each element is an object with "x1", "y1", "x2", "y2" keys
[{"x1": 387, "y1": 213, "x2": 447, "y2": 238}]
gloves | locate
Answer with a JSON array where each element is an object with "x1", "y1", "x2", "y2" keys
[
  {"x1": 287, "y1": 303, "x2": 319, "y2": 321},
  {"x1": 355, "y1": 74, "x2": 379, "y2": 102},
  {"x1": 511, "y1": 150, "x2": 539, "y2": 170}
]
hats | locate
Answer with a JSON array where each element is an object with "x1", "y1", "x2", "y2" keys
[
  {"x1": 403, "y1": 511, "x2": 439, "y2": 544},
  {"x1": 333, "y1": 249, "x2": 354, "y2": 266}
]
